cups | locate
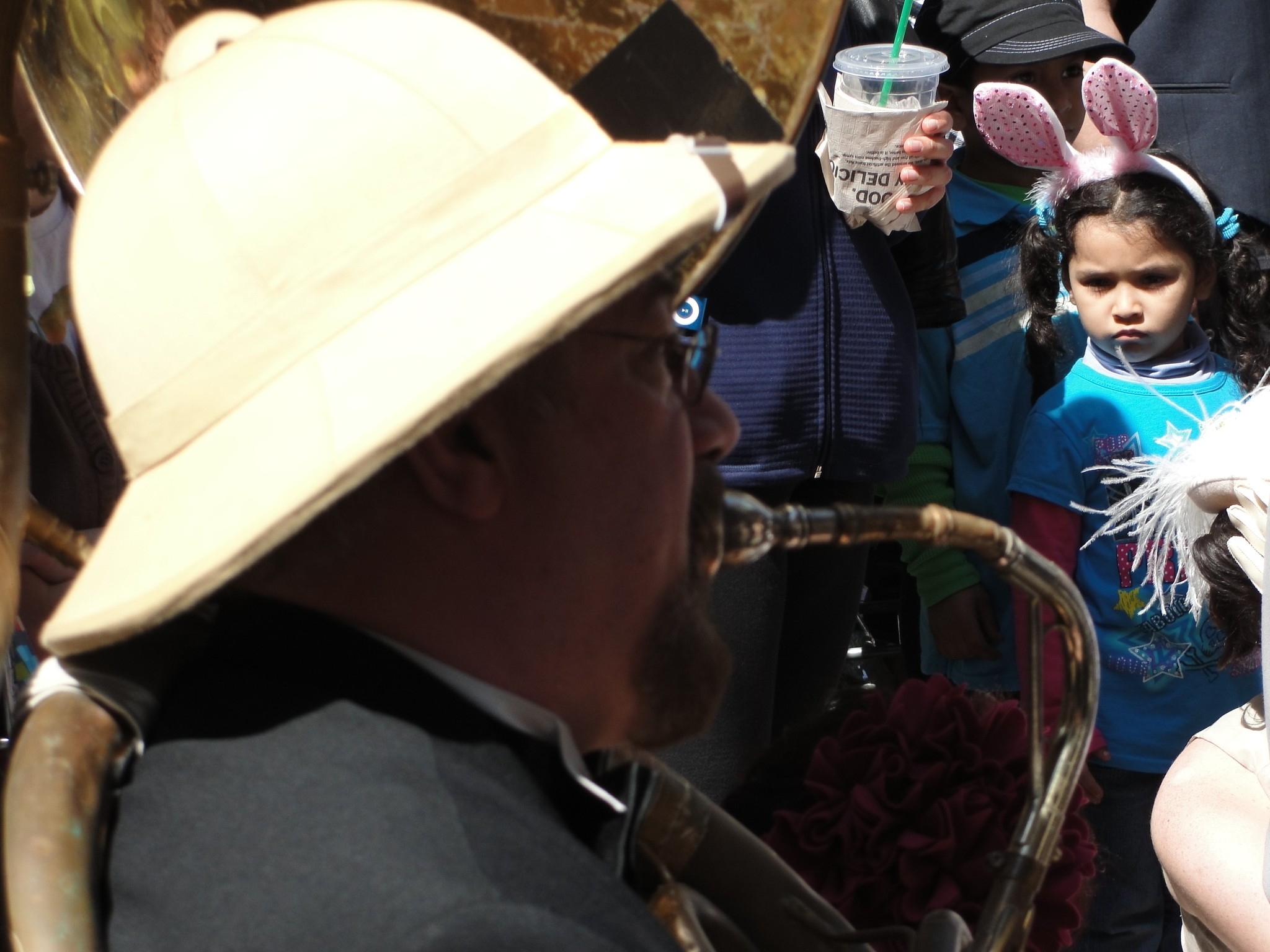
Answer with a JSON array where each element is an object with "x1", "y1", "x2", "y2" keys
[{"x1": 833, "y1": 42, "x2": 951, "y2": 214}]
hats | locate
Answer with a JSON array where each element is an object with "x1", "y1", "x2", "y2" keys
[
  {"x1": 40, "y1": 2, "x2": 797, "y2": 656},
  {"x1": 915, "y1": 0, "x2": 1137, "y2": 80}
]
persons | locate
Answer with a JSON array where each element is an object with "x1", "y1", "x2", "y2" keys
[
  {"x1": 0, "y1": 0, "x2": 1270, "y2": 952},
  {"x1": 40, "y1": 0, "x2": 795, "y2": 951}
]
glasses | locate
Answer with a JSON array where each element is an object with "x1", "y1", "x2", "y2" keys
[{"x1": 568, "y1": 317, "x2": 723, "y2": 402}]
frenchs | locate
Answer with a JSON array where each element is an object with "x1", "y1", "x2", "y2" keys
[{"x1": 0, "y1": 0, "x2": 1103, "y2": 952}]
